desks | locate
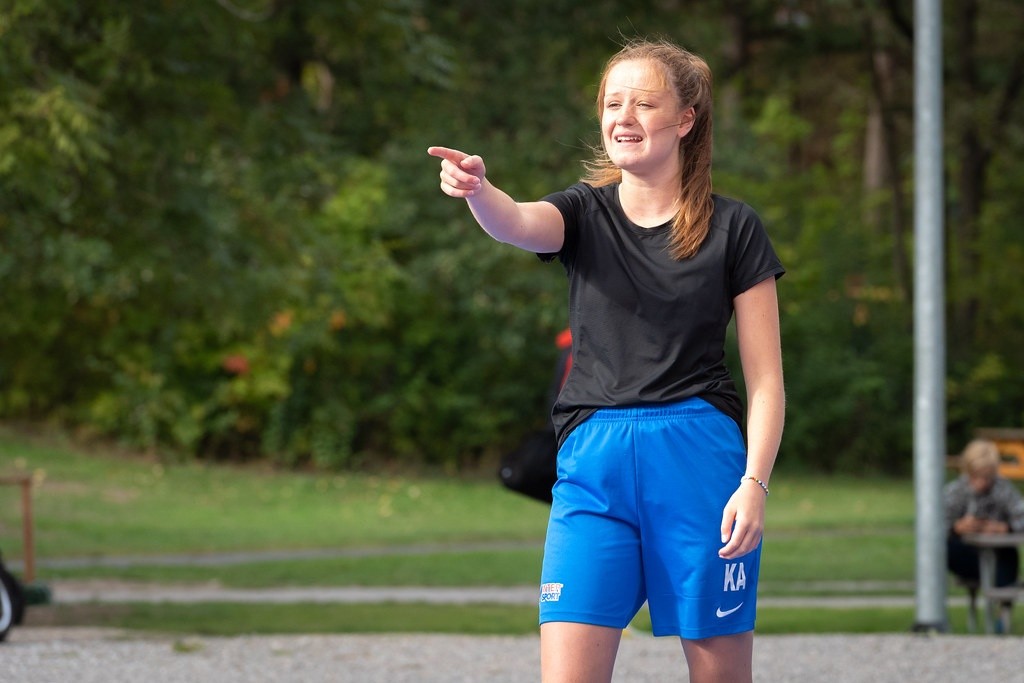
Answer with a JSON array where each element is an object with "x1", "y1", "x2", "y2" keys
[{"x1": 963, "y1": 534, "x2": 1024, "y2": 637}]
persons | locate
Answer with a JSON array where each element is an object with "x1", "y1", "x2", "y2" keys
[
  {"x1": 941, "y1": 438, "x2": 1024, "y2": 634},
  {"x1": 427, "y1": 36, "x2": 786, "y2": 683}
]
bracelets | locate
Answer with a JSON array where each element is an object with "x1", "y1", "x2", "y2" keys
[{"x1": 741, "y1": 476, "x2": 769, "y2": 495}]
